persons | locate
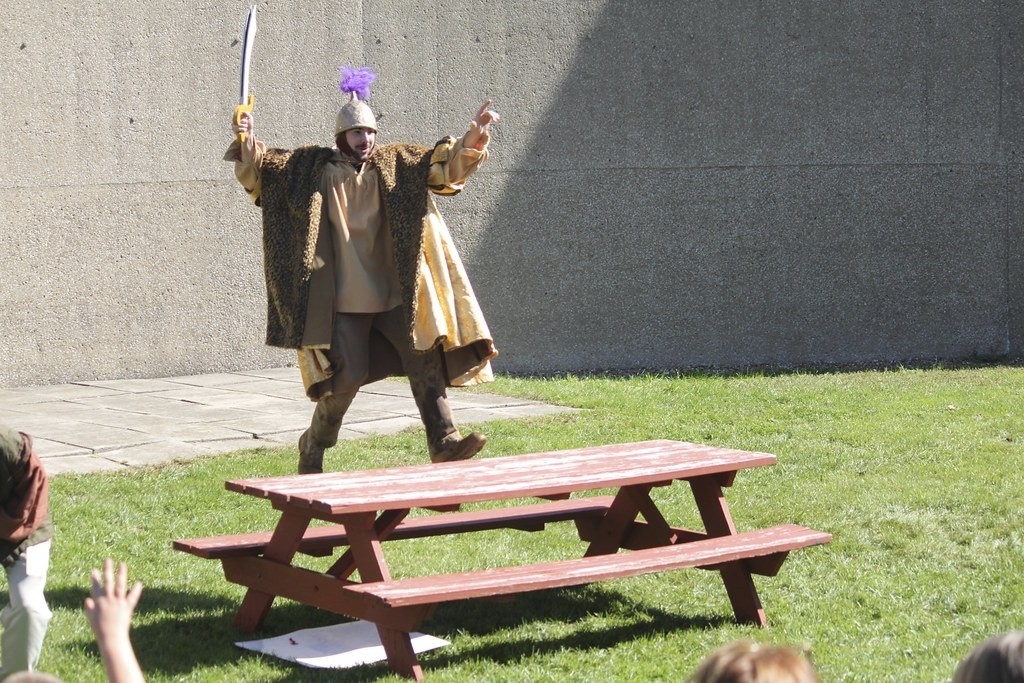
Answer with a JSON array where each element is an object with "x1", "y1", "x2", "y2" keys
[
  {"x1": 223, "y1": 67, "x2": 500, "y2": 476},
  {"x1": 952, "y1": 631, "x2": 1024, "y2": 683},
  {"x1": 0, "y1": 430, "x2": 145, "y2": 683},
  {"x1": 684, "y1": 638, "x2": 821, "y2": 682}
]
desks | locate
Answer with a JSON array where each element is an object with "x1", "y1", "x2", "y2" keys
[{"x1": 172, "y1": 440, "x2": 831, "y2": 683}]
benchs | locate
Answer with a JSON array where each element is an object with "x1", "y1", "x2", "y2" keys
[
  {"x1": 172, "y1": 495, "x2": 616, "y2": 560},
  {"x1": 342, "y1": 523, "x2": 833, "y2": 608}
]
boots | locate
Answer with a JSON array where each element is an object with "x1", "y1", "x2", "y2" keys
[
  {"x1": 400, "y1": 348, "x2": 486, "y2": 464},
  {"x1": 299, "y1": 395, "x2": 353, "y2": 474}
]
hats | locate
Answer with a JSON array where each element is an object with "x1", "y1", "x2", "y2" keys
[{"x1": 334, "y1": 71, "x2": 378, "y2": 137}]
